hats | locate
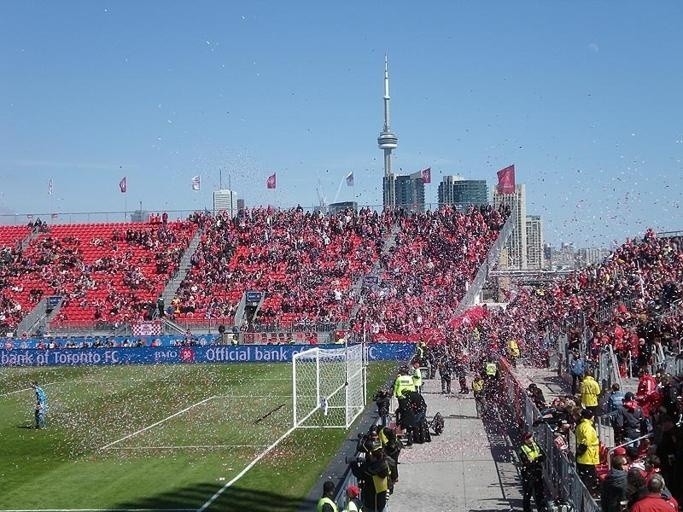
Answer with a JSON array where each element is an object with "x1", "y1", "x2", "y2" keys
[
  {"x1": 524, "y1": 431, "x2": 533, "y2": 440},
  {"x1": 348, "y1": 486, "x2": 359, "y2": 496}
]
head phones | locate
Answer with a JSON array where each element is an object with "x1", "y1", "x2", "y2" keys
[
  {"x1": 627, "y1": 467, "x2": 645, "y2": 486},
  {"x1": 644, "y1": 474, "x2": 665, "y2": 491}
]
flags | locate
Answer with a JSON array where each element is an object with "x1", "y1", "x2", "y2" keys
[
  {"x1": 119, "y1": 176, "x2": 126, "y2": 192},
  {"x1": 497, "y1": 164, "x2": 515, "y2": 195},
  {"x1": 345, "y1": 171, "x2": 354, "y2": 186},
  {"x1": 422, "y1": 168, "x2": 430, "y2": 184},
  {"x1": 266, "y1": 173, "x2": 277, "y2": 189},
  {"x1": 192, "y1": 176, "x2": 201, "y2": 192},
  {"x1": 48, "y1": 179, "x2": 53, "y2": 195}
]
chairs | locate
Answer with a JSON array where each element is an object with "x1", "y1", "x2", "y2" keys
[{"x1": 0, "y1": 219, "x2": 379, "y2": 330}]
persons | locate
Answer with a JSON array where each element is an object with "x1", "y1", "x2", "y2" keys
[
  {"x1": 0, "y1": 201, "x2": 510, "y2": 351},
  {"x1": 416, "y1": 342, "x2": 474, "y2": 394},
  {"x1": 27, "y1": 379, "x2": 46, "y2": 428},
  {"x1": 473, "y1": 228, "x2": 682, "y2": 511},
  {"x1": 314, "y1": 362, "x2": 444, "y2": 511}
]
